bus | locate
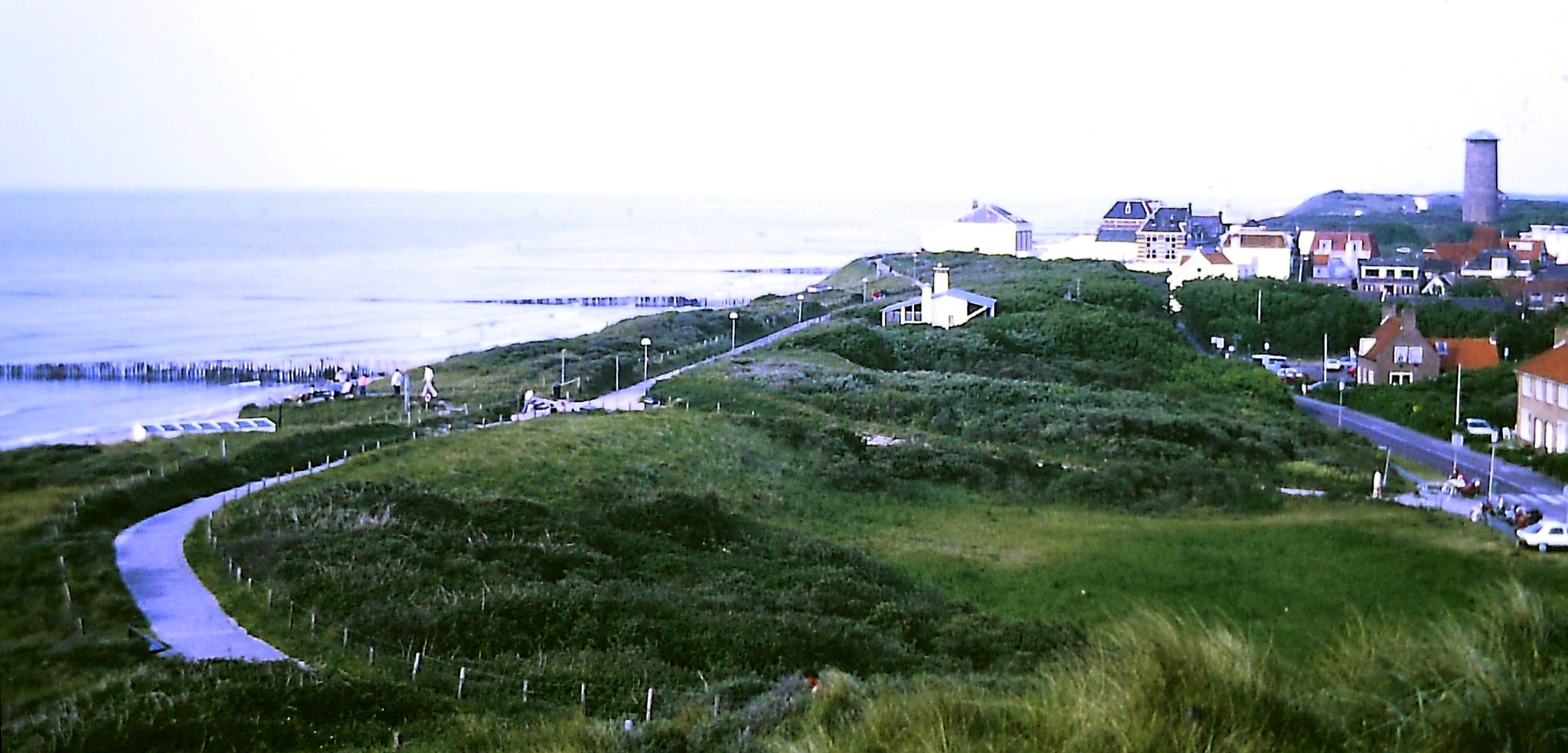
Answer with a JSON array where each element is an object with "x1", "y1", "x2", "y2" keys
[{"x1": 1251, "y1": 355, "x2": 1289, "y2": 369}]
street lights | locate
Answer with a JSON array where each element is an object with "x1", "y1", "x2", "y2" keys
[
  {"x1": 1378, "y1": 445, "x2": 1391, "y2": 486},
  {"x1": 912, "y1": 253, "x2": 918, "y2": 279},
  {"x1": 1339, "y1": 380, "x2": 1345, "y2": 427},
  {"x1": 729, "y1": 312, "x2": 738, "y2": 349},
  {"x1": 862, "y1": 277, "x2": 868, "y2": 303},
  {"x1": 797, "y1": 294, "x2": 805, "y2": 323},
  {"x1": 641, "y1": 337, "x2": 651, "y2": 381},
  {"x1": 562, "y1": 349, "x2": 567, "y2": 383}
]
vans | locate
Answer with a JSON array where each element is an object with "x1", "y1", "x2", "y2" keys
[{"x1": 1461, "y1": 418, "x2": 1500, "y2": 435}]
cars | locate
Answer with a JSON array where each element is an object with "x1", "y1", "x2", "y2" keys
[
  {"x1": 1306, "y1": 380, "x2": 1354, "y2": 393},
  {"x1": 872, "y1": 291, "x2": 884, "y2": 298},
  {"x1": 1326, "y1": 356, "x2": 1358, "y2": 378},
  {"x1": 1504, "y1": 503, "x2": 1568, "y2": 552}
]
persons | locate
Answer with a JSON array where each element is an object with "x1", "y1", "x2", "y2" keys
[
  {"x1": 420, "y1": 366, "x2": 438, "y2": 398},
  {"x1": 357, "y1": 374, "x2": 367, "y2": 396},
  {"x1": 391, "y1": 369, "x2": 403, "y2": 395},
  {"x1": 331, "y1": 368, "x2": 354, "y2": 397},
  {"x1": 804, "y1": 668, "x2": 822, "y2": 692},
  {"x1": 1373, "y1": 466, "x2": 1383, "y2": 499}
]
